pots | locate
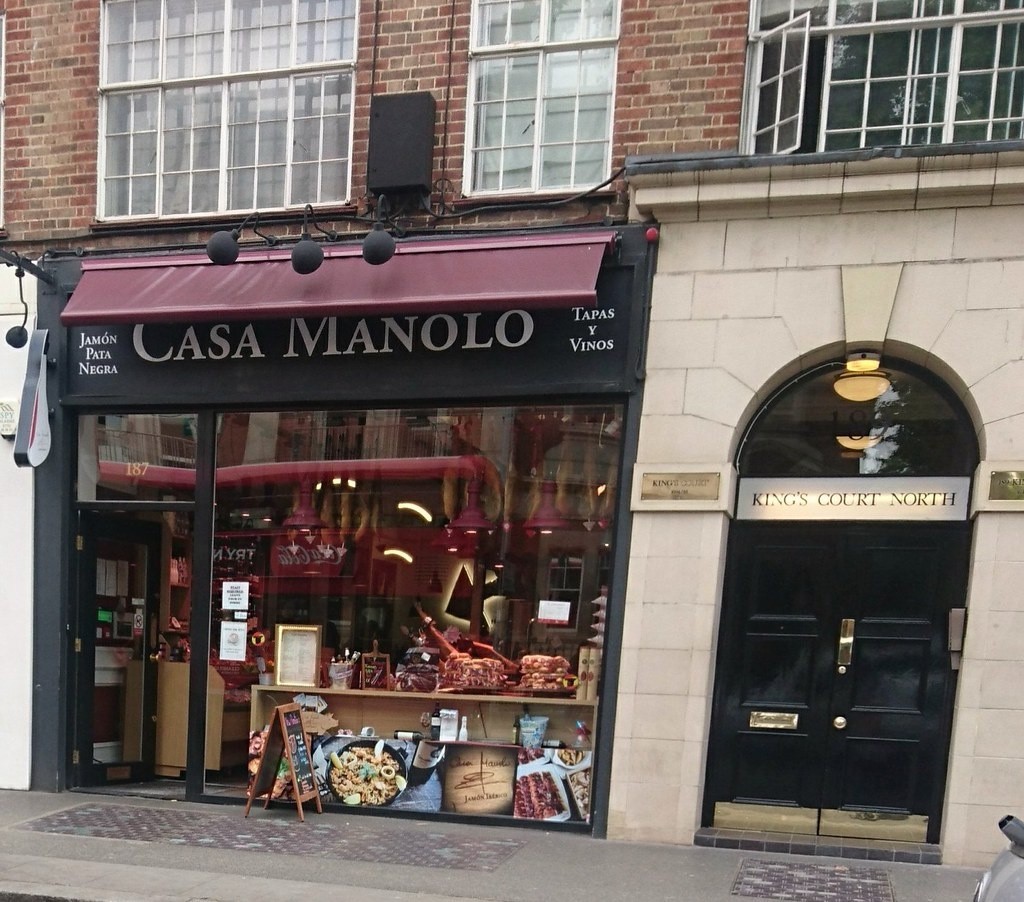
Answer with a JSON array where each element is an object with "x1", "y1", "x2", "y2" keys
[{"x1": 324, "y1": 740, "x2": 408, "y2": 807}]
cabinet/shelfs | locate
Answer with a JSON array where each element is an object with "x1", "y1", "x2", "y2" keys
[{"x1": 92, "y1": 494, "x2": 598, "y2": 821}]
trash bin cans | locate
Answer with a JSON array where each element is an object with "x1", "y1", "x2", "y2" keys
[{"x1": 361, "y1": 653, "x2": 390, "y2": 691}]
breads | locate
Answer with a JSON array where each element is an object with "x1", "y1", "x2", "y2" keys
[{"x1": 516, "y1": 772, "x2": 565, "y2": 821}]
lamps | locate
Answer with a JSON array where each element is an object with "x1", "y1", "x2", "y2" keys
[
  {"x1": 835, "y1": 432, "x2": 882, "y2": 458},
  {"x1": 206, "y1": 211, "x2": 274, "y2": 266},
  {"x1": 521, "y1": 466, "x2": 572, "y2": 534},
  {"x1": 362, "y1": 195, "x2": 405, "y2": 265},
  {"x1": 604, "y1": 417, "x2": 623, "y2": 436},
  {"x1": 291, "y1": 204, "x2": 337, "y2": 275},
  {"x1": 445, "y1": 478, "x2": 498, "y2": 535},
  {"x1": 833, "y1": 349, "x2": 891, "y2": 402},
  {"x1": 5, "y1": 266, "x2": 29, "y2": 349},
  {"x1": 282, "y1": 478, "x2": 329, "y2": 532}
]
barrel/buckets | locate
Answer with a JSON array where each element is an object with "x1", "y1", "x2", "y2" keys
[
  {"x1": 329, "y1": 663, "x2": 354, "y2": 689},
  {"x1": 519, "y1": 717, "x2": 549, "y2": 746}
]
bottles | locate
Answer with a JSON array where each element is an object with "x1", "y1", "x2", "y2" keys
[
  {"x1": 331, "y1": 647, "x2": 361, "y2": 664},
  {"x1": 431, "y1": 701, "x2": 440, "y2": 741},
  {"x1": 459, "y1": 716, "x2": 468, "y2": 741},
  {"x1": 394, "y1": 730, "x2": 431, "y2": 741},
  {"x1": 409, "y1": 739, "x2": 444, "y2": 787},
  {"x1": 512, "y1": 715, "x2": 520, "y2": 744},
  {"x1": 524, "y1": 708, "x2": 530, "y2": 721},
  {"x1": 541, "y1": 740, "x2": 572, "y2": 749}
]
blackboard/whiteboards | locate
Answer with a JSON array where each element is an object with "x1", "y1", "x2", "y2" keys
[{"x1": 248, "y1": 702, "x2": 320, "y2": 803}]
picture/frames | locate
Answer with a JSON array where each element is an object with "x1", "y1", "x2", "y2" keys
[{"x1": 274, "y1": 623, "x2": 323, "y2": 688}]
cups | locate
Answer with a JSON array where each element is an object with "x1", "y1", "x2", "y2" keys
[{"x1": 361, "y1": 727, "x2": 375, "y2": 736}]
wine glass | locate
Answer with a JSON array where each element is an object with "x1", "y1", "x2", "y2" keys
[{"x1": 421, "y1": 712, "x2": 431, "y2": 741}]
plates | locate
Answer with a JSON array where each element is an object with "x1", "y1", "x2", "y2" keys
[
  {"x1": 552, "y1": 749, "x2": 592, "y2": 770},
  {"x1": 358, "y1": 735, "x2": 379, "y2": 738},
  {"x1": 514, "y1": 764, "x2": 571, "y2": 823},
  {"x1": 335, "y1": 735, "x2": 356, "y2": 738},
  {"x1": 529, "y1": 748, "x2": 554, "y2": 764}
]
